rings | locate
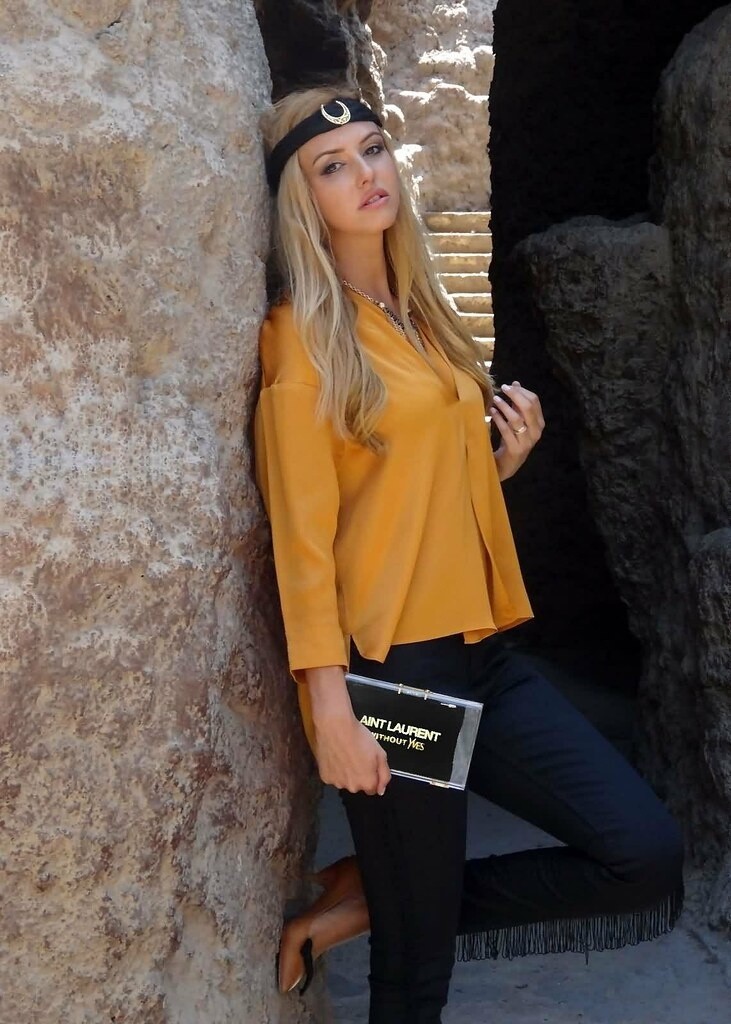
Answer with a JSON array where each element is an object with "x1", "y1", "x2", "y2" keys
[{"x1": 513, "y1": 426, "x2": 528, "y2": 435}]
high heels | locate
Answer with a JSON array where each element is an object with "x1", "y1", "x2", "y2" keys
[{"x1": 276, "y1": 856, "x2": 371, "y2": 994}]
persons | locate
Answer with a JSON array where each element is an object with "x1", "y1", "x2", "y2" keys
[{"x1": 259, "y1": 89, "x2": 682, "y2": 1024}]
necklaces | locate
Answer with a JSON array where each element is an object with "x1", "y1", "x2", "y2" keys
[{"x1": 339, "y1": 279, "x2": 422, "y2": 343}]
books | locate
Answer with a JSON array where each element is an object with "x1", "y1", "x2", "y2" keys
[{"x1": 344, "y1": 672, "x2": 484, "y2": 791}]
